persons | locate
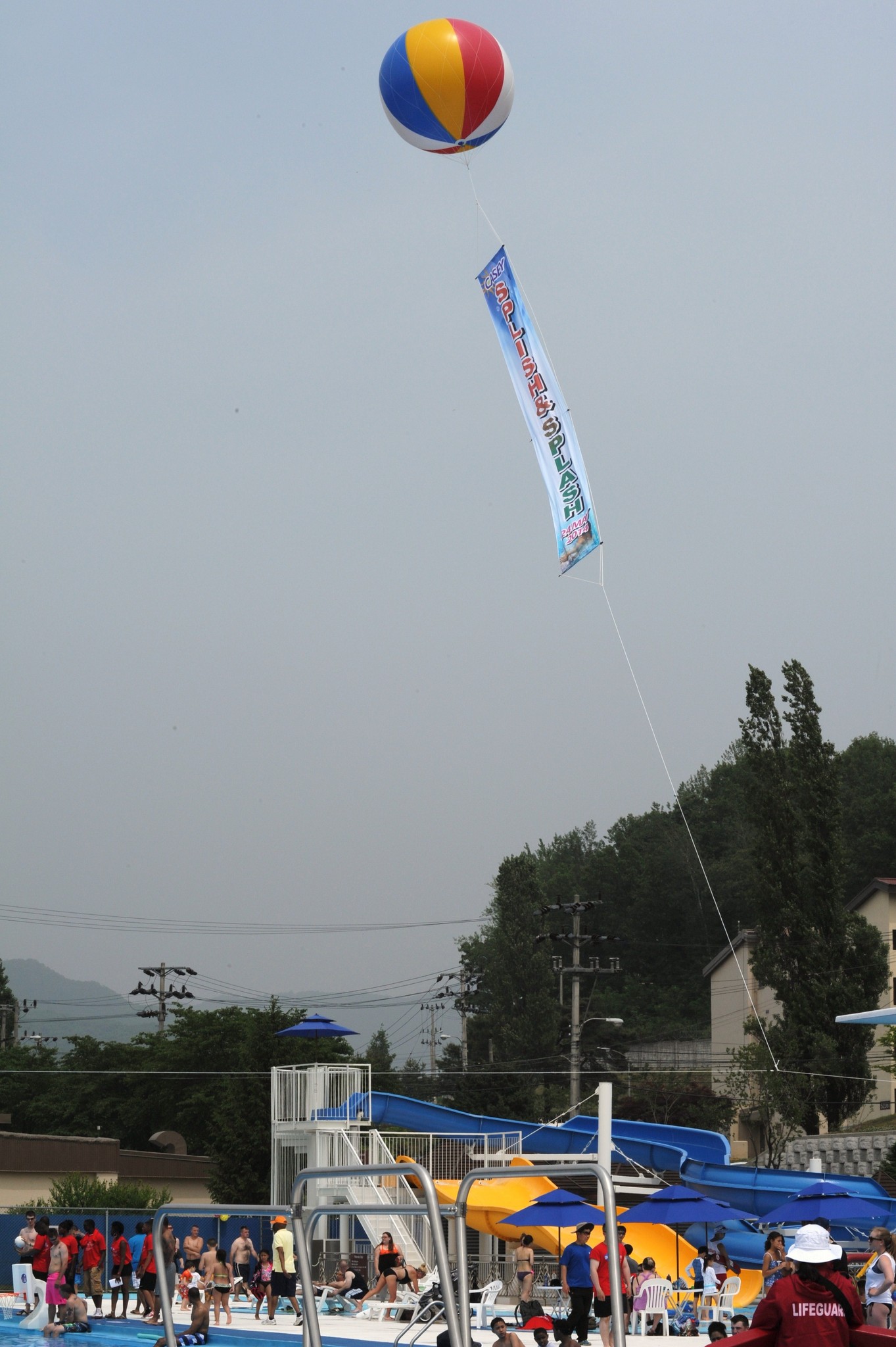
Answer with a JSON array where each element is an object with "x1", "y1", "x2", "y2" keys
[{"x1": 15, "y1": 1210, "x2": 896, "y2": 1347}]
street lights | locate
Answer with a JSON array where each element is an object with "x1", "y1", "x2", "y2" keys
[
  {"x1": 596, "y1": 1046, "x2": 631, "y2": 1098},
  {"x1": 570, "y1": 1017, "x2": 625, "y2": 1120},
  {"x1": 440, "y1": 1034, "x2": 468, "y2": 1077},
  {"x1": 12, "y1": 1035, "x2": 42, "y2": 1047}
]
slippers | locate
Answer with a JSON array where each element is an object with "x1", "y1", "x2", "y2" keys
[
  {"x1": 646, "y1": 1320, "x2": 653, "y2": 1326},
  {"x1": 130, "y1": 1310, "x2": 140, "y2": 1314},
  {"x1": 144, "y1": 1321, "x2": 157, "y2": 1326},
  {"x1": 116, "y1": 1315, "x2": 127, "y2": 1319},
  {"x1": 647, "y1": 1330, "x2": 653, "y2": 1335},
  {"x1": 27, "y1": 1310, "x2": 33, "y2": 1316},
  {"x1": 104, "y1": 1313, "x2": 116, "y2": 1319},
  {"x1": 16, "y1": 1309, "x2": 29, "y2": 1315}
]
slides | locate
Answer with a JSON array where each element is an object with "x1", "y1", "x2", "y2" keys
[
  {"x1": 340, "y1": 1089, "x2": 896, "y2": 1275},
  {"x1": 397, "y1": 1151, "x2": 765, "y2": 1311}
]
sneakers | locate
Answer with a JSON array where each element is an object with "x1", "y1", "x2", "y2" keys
[
  {"x1": 293, "y1": 1315, "x2": 303, "y2": 1326},
  {"x1": 91, "y1": 1310, "x2": 103, "y2": 1318},
  {"x1": 146, "y1": 1310, "x2": 155, "y2": 1319},
  {"x1": 261, "y1": 1316, "x2": 276, "y2": 1325},
  {"x1": 330, "y1": 1307, "x2": 344, "y2": 1313},
  {"x1": 95, "y1": 1308, "x2": 103, "y2": 1319}
]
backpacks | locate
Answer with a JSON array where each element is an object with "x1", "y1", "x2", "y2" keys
[{"x1": 520, "y1": 1299, "x2": 545, "y2": 1326}]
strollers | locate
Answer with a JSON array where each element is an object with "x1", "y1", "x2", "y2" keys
[{"x1": 417, "y1": 1261, "x2": 481, "y2": 1323}]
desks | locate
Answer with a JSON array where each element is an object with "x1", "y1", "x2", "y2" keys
[
  {"x1": 663, "y1": 1288, "x2": 703, "y2": 1326},
  {"x1": 537, "y1": 1286, "x2": 571, "y2": 1320}
]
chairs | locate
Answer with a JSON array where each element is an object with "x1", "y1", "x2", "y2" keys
[
  {"x1": 469, "y1": 1280, "x2": 503, "y2": 1329},
  {"x1": 171, "y1": 1274, "x2": 243, "y2": 1306},
  {"x1": 632, "y1": 1278, "x2": 673, "y2": 1336},
  {"x1": 249, "y1": 1278, "x2": 351, "y2": 1312},
  {"x1": 697, "y1": 1276, "x2": 741, "y2": 1322},
  {"x1": 366, "y1": 1264, "x2": 441, "y2": 1322}
]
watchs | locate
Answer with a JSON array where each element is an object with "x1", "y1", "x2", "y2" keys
[{"x1": 96, "y1": 1266, "x2": 100, "y2": 1270}]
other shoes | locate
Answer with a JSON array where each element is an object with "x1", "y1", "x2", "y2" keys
[
  {"x1": 141, "y1": 1309, "x2": 151, "y2": 1318},
  {"x1": 578, "y1": 1340, "x2": 591, "y2": 1345},
  {"x1": 625, "y1": 1332, "x2": 631, "y2": 1335}
]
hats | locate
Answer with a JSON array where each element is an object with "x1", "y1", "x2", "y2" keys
[
  {"x1": 812, "y1": 1217, "x2": 830, "y2": 1230},
  {"x1": 270, "y1": 1216, "x2": 288, "y2": 1224},
  {"x1": 571, "y1": 1222, "x2": 594, "y2": 1233},
  {"x1": 785, "y1": 1224, "x2": 842, "y2": 1263}
]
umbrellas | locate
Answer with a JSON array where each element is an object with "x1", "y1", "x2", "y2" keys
[
  {"x1": 617, "y1": 1186, "x2": 760, "y2": 1305},
  {"x1": 494, "y1": 1187, "x2": 608, "y2": 1286},
  {"x1": 701, "y1": 1197, "x2": 761, "y2": 1248},
  {"x1": 273, "y1": 1013, "x2": 361, "y2": 1063},
  {"x1": 755, "y1": 1182, "x2": 896, "y2": 1224}
]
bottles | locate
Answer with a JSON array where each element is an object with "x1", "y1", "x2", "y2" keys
[
  {"x1": 666, "y1": 1274, "x2": 671, "y2": 1283},
  {"x1": 544, "y1": 1274, "x2": 548, "y2": 1287},
  {"x1": 551, "y1": 1270, "x2": 556, "y2": 1279}
]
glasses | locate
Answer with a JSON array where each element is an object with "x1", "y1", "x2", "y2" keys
[
  {"x1": 869, "y1": 1236, "x2": 882, "y2": 1243},
  {"x1": 26, "y1": 1218, "x2": 34, "y2": 1220},
  {"x1": 580, "y1": 1231, "x2": 591, "y2": 1235},
  {"x1": 618, "y1": 1232, "x2": 625, "y2": 1235},
  {"x1": 381, "y1": 1236, "x2": 389, "y2": 1238},
  {"x1": 167, "y1": 1227, "x2": 172, "y2": 1230}
]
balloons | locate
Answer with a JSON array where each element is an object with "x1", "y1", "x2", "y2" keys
[
  {"x1": 220, "y1": 1215, "x2": 228, "y2": 1221},
  {"x1": 377, "y1": 18, "x2": 515, "y2": 155}
]
